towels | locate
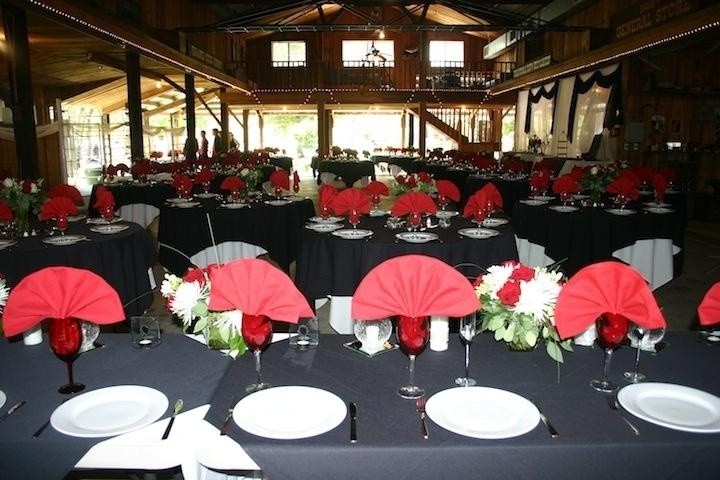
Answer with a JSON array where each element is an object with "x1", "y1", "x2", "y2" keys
[
  {"x1": 697, "y1": 283, "x2": 720, "y2": 326},
  {"x1": 3, "y1": 266, "x2": 124, "y2": 352},
  {"x1": 207, "y1": 258, "x2": 316, "y2": 345},
  {"x1": 555, "y1": 261, "x2": 667, "y2": 343},
  {"x1": 351, "y1": 254, "x2": 482, "y2": 346}
]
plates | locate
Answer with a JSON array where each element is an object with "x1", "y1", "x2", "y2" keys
[
  {"x1": 618, "y1": 383, "x2": 720, "y2": 434},
  {"x1": 232, "y1": 383, "x2": 347, "y2": 440},
  {"x1": 424, "y1": 386, "x2": 541, "y2": 440},
  {"x1": 0, "y1": 214, "x2": 131, "y2": 250},
  {"x1": 303, "y1": 210, "x2": 510, "y2": 244},
  {"x1": 519, "y1": 189, "x2": 681, "y2": 216},
  {"x1": 49, "y1": 385, "x2": 170, "y2": 440},
  {"x1": 165, "y1": 191, "x2": 306, "y2": 209}
]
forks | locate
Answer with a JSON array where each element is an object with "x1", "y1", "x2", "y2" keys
[
  {"x1": 415, "y1": 400, "x2": 430, "y2": 440},
  {"x1": 605, "y1": 395, "x2": 640, "y2": 435},
  {"x1": 220, "y1": 402, "x2": 237, "y2": 436}
]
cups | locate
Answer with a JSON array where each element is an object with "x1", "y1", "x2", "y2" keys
[
  {"x1": 80, "y1": 320, "x2": 100, "y2": 348},
  {"x1": 204, "y1": 317, "x2": 233, "y2": 349},
  {"x1": 625, "y1": 327, "x2": 666, "y2": 348},
  {"x1": 129, "y1": 316, "x2": 161, "y2": 350},
  {"x1": 286, "y1": 310, "x2": 320, "y2": 350},
  {"x1": 354, "y1": 318, "x2": 395, "y2": 348}
]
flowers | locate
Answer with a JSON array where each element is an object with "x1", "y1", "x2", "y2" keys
[
  {"x1": 159, "y1": 214, "x2": 249, "y2": 358},
  {"x1": 452, "y1": 259, "x2": 570, "y2": 384}
]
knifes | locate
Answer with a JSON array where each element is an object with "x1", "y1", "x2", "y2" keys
[
  {"x1": 1, "y1": 399, "x2": 29, "y2": 421},
  {"x1": 162, "y1": 399, "x2": 184, "y2": 439},
  {"x1": 346, "y1": 401, "x2": 359, "y2": 445}
]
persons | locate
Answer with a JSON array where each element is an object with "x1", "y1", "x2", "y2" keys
[{"x1": 184, "y1": 129, "x2": 240, "y2": 162}]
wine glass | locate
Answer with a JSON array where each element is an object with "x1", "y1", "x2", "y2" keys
[
  {"x1": 623, "y1": 317, "x2": 650, "y2": 382},
  {"x1": 240, "y1": 312, "x2": 273, "y2": 392},
  {"x1": 109, "y1": 174, "x2": 147, "y2": 185},
  {"x1": 590, "y1": 313, "x2": 628, "y2": 392},
  {"x1": 394, "y1": 315, "x2": 430, "y2": 398},
  {"x1": 47, "y1": 315, "x2": 86, "y2": 394},
  {"x1": 454, "y1": 311, "x2": 478, "y2": 387},
  {"x1": 529, "y1": 178, "x2": 676, "y2": 212},
  {"x1": 176, "y1": 181, "x2": 300, "y2": 204},
  {"x1": 0, "y1": 207, "x2": 115, "y2": 236},
  {"x1": 319, "y1": 193, "x2": 494, "y2": 232}
]
